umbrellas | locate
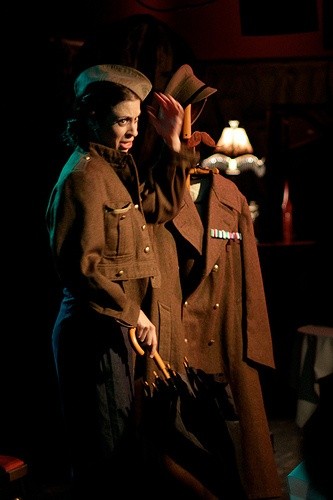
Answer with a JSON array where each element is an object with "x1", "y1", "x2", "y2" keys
[{"x1": 130, "y1": 327, "x2": 247, "y2": 500}]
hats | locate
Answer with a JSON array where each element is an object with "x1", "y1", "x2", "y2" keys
[
  {"x1": 163, "y1": 63, "x2": 218, "y2": 110},
  {"x1": 72, "y1": 63, "x2": 153, "y2": 104}
]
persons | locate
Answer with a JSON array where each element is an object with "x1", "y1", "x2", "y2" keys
[{"x1": 46, "y1": 65, "x2": 198, "y2": 500}]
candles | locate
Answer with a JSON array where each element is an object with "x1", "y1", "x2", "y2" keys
[{"x1": 281, "y1": 182, "x2": 293, "y2": 242}]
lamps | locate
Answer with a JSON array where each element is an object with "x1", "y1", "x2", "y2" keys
[{"x1": 202, "y1": 121, "x2": 262, "y2": 177}]
